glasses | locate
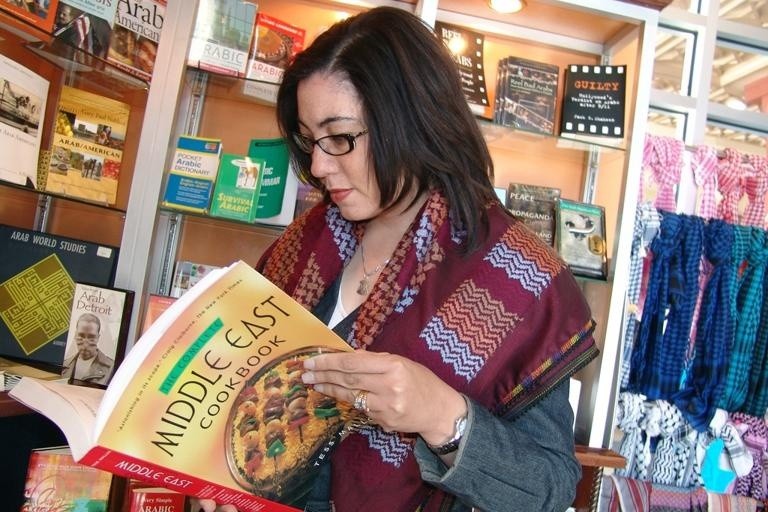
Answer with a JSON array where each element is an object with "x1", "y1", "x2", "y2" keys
[
  {"x1": 74, "y1": 331, "x2": 99, "y2": 344},
  {"x1": 292, "y1": 125, "x2": 369, "y2": 156}
]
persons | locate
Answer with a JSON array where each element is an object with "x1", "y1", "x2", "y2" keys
[{"x1": 189, "y1": 5, "x2": 603, "y2": 512}]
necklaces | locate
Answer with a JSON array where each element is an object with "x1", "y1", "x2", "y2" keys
[{"x1": 355, "y1": 236, "x2": 394, "y2": 295}]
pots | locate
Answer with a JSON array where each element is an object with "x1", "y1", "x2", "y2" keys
[{"x1": 221, "y1": 344, "x2": 357, "y2": 502}]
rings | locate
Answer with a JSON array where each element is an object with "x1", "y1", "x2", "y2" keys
[
  {"x1": 350, "y1": 390, "x2": 369, "y2": 415},
  {"x1": 359, "y1": 412, "x2": 375, "y2": 426}
]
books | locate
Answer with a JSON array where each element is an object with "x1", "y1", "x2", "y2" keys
[
  {"x1": 162, "y1": 136, "x2": 324, "y2": 227},
  {"x1": 21, "y1": 444, "x2": 113, "y2": 512},
  {"x1": 186, "y1": 0, "x2": 307, "y2": 107},
  {"x1": 434, "y1": 20, "x2": 490, "y2": 117},
  {"x1": 492, "y1": 183, "x2": 608, "y2": 279},
  {"x1": 132, "y1": 487, "x2": 184, "y2": 512},
  {"x1": 493, "y1": 57, "x2": 628, "y2": 150},
  {"x1": 0, "y1": 55, "x2": 130, "y2": 207},
  {"x1": 122, "y1": 476, "x2": 152, "y2": 512},
  {"x1": 0, "y1": 0, "x2": 168, "y2": 89},
  {"x1": 1, "y1": 223, "x2": 223, "y2": 393},
  {"x1": 4, "y1": 257, "x2": 364, "y2": 512}
]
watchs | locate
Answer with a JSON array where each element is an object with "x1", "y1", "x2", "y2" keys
[{"x1": 420, "y1": 407, "x2": 469, "y2": 456}]
